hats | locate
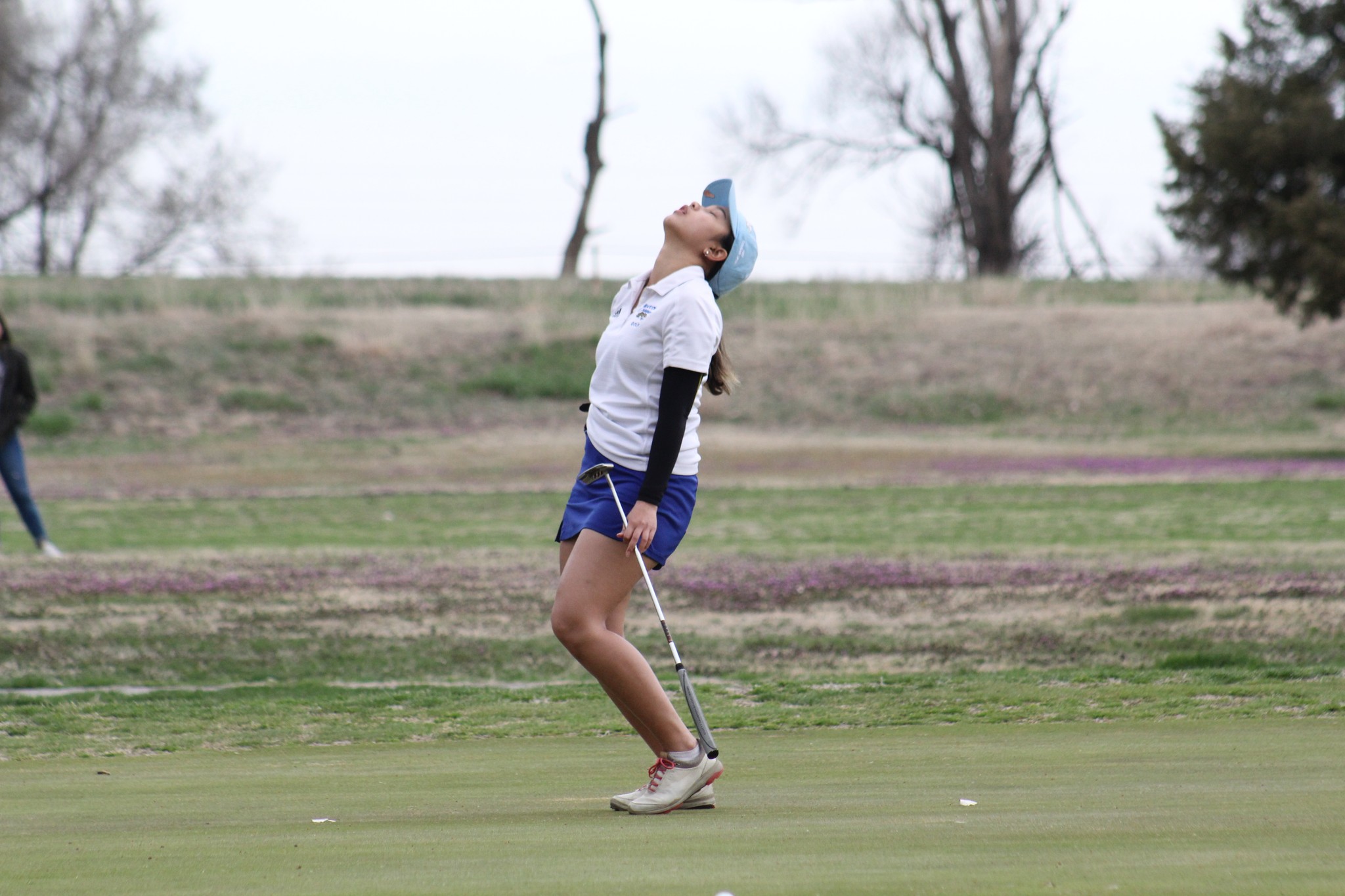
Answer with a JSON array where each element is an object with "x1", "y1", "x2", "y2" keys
[{"x1": 702, "y1": 179, "x2": 758, "y2": 297}]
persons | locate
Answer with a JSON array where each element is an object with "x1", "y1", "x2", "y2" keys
[
  {"x1": 0, "y1": 311, "x2": 61, "y2": 559},
  {"x1": 549, "y1": 177, "x2": 759, "y2": 816}
]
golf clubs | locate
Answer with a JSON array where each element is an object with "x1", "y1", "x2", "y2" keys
[{"x1": 578, "y1": 463, "x2": 723, "y2": 762}]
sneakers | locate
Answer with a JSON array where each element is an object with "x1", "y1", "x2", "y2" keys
[
  {"x1": 627, "y1": 738, "x2": 724, "y2": 814},
  {"x1": 611, "y1": 783, "x2": 716, "y2": 811}
]
